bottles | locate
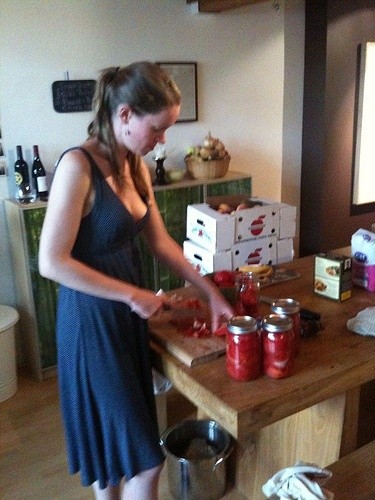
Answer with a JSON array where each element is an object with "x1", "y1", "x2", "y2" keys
[
  {"x1": 14, "y1": 146, "x2": 31, "y2": 196},
  {"x1": 235, "y1": 272, "x2": 261, "y2": 320},
  {"x1": 271, "y1": 298, "x2": 302, "y2": 356},
  {"x1": 225, "y1": 315, "x2": 263, "y2": 382},
  {"x1": 260, "y1": 314, "x2": 299, "y2": 379},
  {"x1": 31, "y1": 145, "x2": 48, "y2": 198}
]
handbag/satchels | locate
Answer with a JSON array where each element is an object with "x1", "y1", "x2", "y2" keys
[{"x1": 261, "y1": 462, "x2": 334, "y2": 500}]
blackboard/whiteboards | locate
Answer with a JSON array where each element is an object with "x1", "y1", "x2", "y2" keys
[{"x1": 52, "y1": 80, "x2": 96, "y2": 112}]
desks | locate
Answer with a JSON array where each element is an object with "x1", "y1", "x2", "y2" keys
[{"x1": 147, "y1": 247, "x2": 375, "y2": 500}]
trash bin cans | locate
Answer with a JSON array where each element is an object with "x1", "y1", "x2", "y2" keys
[{"x1": 0, "y1": 304, "x2": 20, "y2": 403}]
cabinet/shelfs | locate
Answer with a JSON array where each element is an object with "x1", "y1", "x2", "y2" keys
[{"x1": 6, "y1": 170, "x2": 252, "y2": 382}]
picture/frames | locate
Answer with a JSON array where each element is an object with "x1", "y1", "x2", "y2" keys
[{"x1": 158, "y1": 62, "x2": 198, "y2": 122}]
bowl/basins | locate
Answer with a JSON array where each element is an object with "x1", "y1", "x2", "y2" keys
[{"x1": 185, "y1": 158, "x2": 230, "y2": 180}]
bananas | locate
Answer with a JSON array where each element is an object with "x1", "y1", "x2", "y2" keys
[{"x1": 237, "y1": 260, "x2": 273, "y2": 278}]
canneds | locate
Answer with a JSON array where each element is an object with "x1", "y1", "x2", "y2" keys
[
  {"x1": 234, "y1": 272, "x2": 261, "y2": 317},
  {"x1": 225, "y1": 297, "x2": 300, "y2": 382}
]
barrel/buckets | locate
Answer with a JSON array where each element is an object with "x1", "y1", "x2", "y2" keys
[{"x1": 159, "y1": 418, "x2": 236, "y2": 500}]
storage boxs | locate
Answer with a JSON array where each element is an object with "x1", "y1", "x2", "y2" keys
[
  {"x1": 183, "y1": 198, "x2": 296, "y2": 287},
  {"x1": 314, "y1": 251, "x2": 351, "y2": 303}
]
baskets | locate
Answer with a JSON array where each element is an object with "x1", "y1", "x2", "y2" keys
[{"x1": 183, "y1": 152, "x2": 231, "y2": 179}]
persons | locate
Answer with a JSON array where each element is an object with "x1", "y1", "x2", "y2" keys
[{"x1": 38, "y1": 60, "x2": 236, "y2": 500}]
